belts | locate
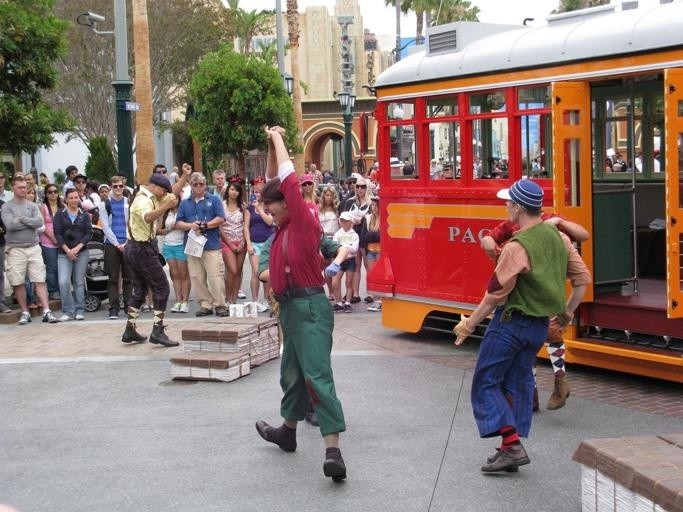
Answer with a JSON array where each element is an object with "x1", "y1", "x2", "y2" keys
[{"x1": 273, "y1": 286, "x2": 324, "y2": 302}]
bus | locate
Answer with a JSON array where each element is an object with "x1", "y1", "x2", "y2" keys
[{"x1": 367, "y1": 1, "x2": 682, "y2": 386}]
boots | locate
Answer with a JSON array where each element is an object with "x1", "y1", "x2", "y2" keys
[
  {"x1": 122, "y1": 325, "x2": 147, "y2": 342},
  {"x1": 546, "y1": 374, "x2": 570, "y2": 410},
  {"x1": 148, "y1": 325, "x2": 178, "y2": 346}
]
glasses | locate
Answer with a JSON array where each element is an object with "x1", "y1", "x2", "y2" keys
[
  {"x1": 77, "y1": 179, "x2": 85, "y2": 184},
  {"x1": 193, "y1": 183, "x2": 204, "y2": 187},
  {"x1": 156, "y1": 170, "x2": 167, "y2": 174},
  {"x1": 113, "y1": 185, "x2": 123, "y2": 189},
  {"x1": 356, "y1": 185, "x2": 366, "y2": 189},
  {"x1": 49, "y1": 190, "x2": 58, "y2": 194}
]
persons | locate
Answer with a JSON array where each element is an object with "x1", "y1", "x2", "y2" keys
[
  {"x1": 452, "y1": 176, "x2": 594, "y2": 475},
  {"x1": 390, "y1": 149, "x2": 661, "y2": 180},
  {"x1": 253, "y1": 125, "x2": 350, "y2": 481},
  {"x1": 1, "y1": 127, "x2": 379, "y2": 345},
  {"x1": 479, "y1": 211, "x2": 590, "y2": 412}
]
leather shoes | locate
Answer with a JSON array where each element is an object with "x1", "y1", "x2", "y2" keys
[
  {"x1": 256, "y1": 420, "x2": 297, "y2": 452},
  {"x1": 323, "y1": 447, "x2": 346, "y2": 481}
]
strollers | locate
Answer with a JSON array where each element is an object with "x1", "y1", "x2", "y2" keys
[{"x1": 81, "y1": 225, "x2": 127, "y2": 313}]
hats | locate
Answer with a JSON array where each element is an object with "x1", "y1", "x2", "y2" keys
[
  {"x1": 390, "y1": 157, "x2": 405, "y2": 168},
  {"x1": 339, "y1": 211, "x2": 353, "y2": 220},
  {"x1": 348, "y1": 172, "x2": 360, "y2": 180},
  {"x1": 301, "y1": 175, "x2": 314, "y2": 184},
  {"x1": 73, "y1": 174, "x2": 87, "y2": 180},
  {"x1": 150, "y1": 173, "x2": 172, "y2": 193},
  {"x1": 496, "y1": 179, "x2": 543, "y2": 209},
  {"x1": 258, "y1": 178, "x2": 284, "y2": 202}
]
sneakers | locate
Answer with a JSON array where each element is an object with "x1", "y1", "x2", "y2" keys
[
  {"x1": 253, "y1": 299, "x2": 271, "y2": 312},
  {"x1": 0, "y1": 302, "x2": 12, "y2": 313},
  {"x1": 109, "y1": 294, "x2": 188, "y2": 319},
  {"x1": 238, "y1": 289, "x2": 246, "y2": 299},
  {"x1": 41, "y1": 310, "x2": 83, "y2": 323},
  {"x1": 305, "y1": 409, "x2": 319, "y2": 426},
  {"x1": 327, "y1": 294, "x2": 381, "y2": 312},
  {"x1": 18, "y1": 313, "x2": 32, "y2": 324},
  {"x1": 196, "y1": 307, "x2": 214, "y2": 316},
  {"x1": 215, "y1": 306, "x2": 228, "y2": 317},
  {"x1": 481, "y1": 445, "x2": 530, "y2": 472},
  {"x1": 28, "y1": 303, "x2": 39, "y2": 309},
  {"x1": 50, "y1": 294, "x2": 61, "y2": 300}
]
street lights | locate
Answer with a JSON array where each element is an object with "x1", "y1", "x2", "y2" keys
[
  {"x1": 391, "y1": 104, "x2": 404, "y2": 162},
  {"x1": 278, "y1": 73, "x2": 295, "y2": 96},
  {"x1": 336, "y1": 93, "x2": 356, "y2": 179}
]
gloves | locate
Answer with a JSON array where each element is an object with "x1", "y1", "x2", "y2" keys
[
  {"x1": 325, "y1": 261, "x2": 341, "y2": 277},
  {"x1": 551, "y1": 309, "x2": 572, "y2": 333},
  {"x1": 453, "y1": 313, "x2": 473, "y2": 346}
]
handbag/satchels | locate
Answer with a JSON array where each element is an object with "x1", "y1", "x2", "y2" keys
[
  {"x1": 85, "y1": 207, "x2": 99, "y2": 224},
  {"x1": 63, "y1": 225, "x2": 85, "y2": 249}
]
